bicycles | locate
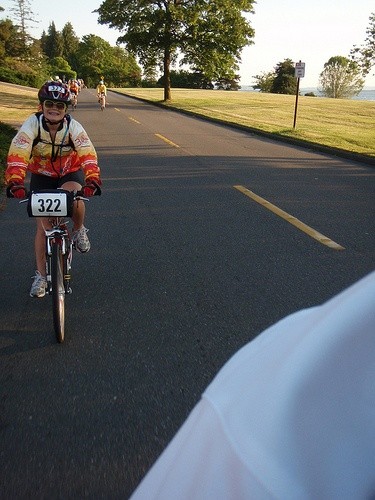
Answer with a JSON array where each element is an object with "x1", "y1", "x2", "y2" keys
[
  {"x1": 96, "y1": 94, "x2": 104, "y2": 111},
  {"x1": 10, "y1": 189, "x2": 102, "y2": 344}
]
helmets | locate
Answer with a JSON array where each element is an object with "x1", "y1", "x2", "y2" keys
[
  {"x1": 38, "y1": 81, "x2": 71, "y2": 108},
  {"x1": 99, "y1": 81, "x2": 103, "y2": 84}
]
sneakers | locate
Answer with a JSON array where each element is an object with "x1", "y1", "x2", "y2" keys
[
  {"x1": 71, "y1": 223, "x2": 91, "y2": 254},
  {"x1": 29, "y1": 270, "x2": 48, "y2": 297}
]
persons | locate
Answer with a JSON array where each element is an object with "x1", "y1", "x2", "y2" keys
[
  {"x1": 58, "y1": 78, "x2": 107, "y2": 110},
  {"x1": 6, "y1": 82, "x2": 102, "y2": 297}
]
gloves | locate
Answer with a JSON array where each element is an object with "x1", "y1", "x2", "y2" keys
[
  {"x1": 9, "y1": 185, "x2": 25, "y2": 199},
  {"x1": 81, "y1": 183, "x2": 97, "y2": 197}
]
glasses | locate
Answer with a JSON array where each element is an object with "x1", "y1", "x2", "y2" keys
[{"x1": 42, "y1": 100, "x2": 67, "y2": 111}]
